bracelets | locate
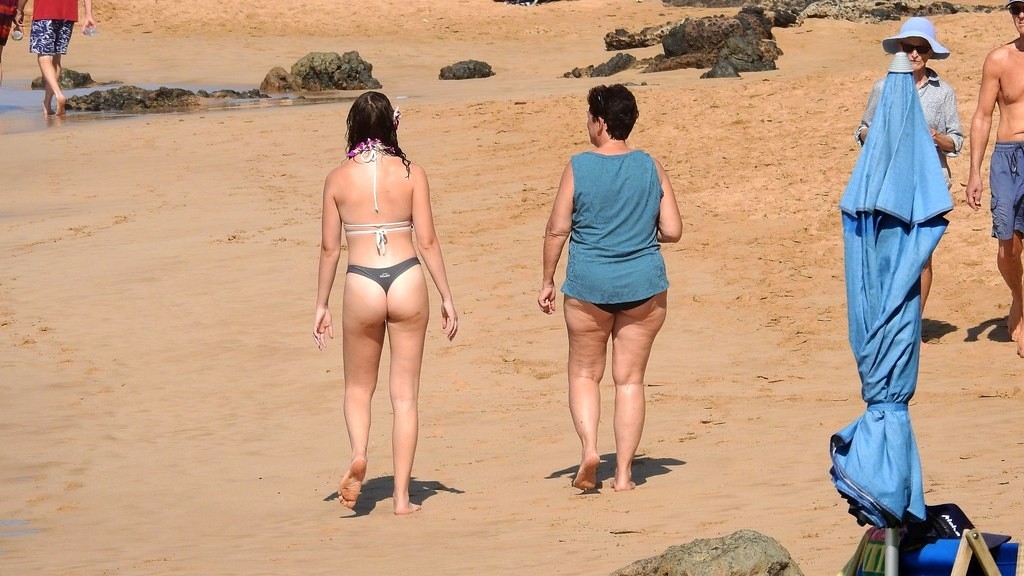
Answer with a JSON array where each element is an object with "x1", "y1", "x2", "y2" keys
[{"x1": 17, "y1": 9, "x2": 23, "y2": 14}]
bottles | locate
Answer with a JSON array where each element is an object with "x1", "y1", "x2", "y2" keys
[
  {"x1": 83, "y1": 28, "x2": 96, "y2": 36},
  {"x1": 12, "y1": 30, "x2": 23, "y2": 41}
]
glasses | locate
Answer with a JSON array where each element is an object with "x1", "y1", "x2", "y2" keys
[
  {"x1": 900, "y1": 41, "x2": 932, "y2": 55},
  {"x1": 1009, "y1": 7, "x2": 1024, "y2": 15}
]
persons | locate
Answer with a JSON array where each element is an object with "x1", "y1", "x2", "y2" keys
[
  {"x1": 537, "y1": 84, "x2": 682, "y2": 492},
  {"x1": 313, "y1": 91, "x2": 458, "y2": 515},
  {"x1": 855, "y1": 17, "x2": 964, "y2": 317},
  {"x1": 967, "y1": 0, "x2": 1024, "y2": 359},
  {"x1": 15, "y1": 0, "x2": 96, "y2": 116},
  {"x1": 0, "y1": 0, "x2": 25, "y2": 88}
]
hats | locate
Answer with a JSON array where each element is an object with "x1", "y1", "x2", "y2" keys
[
  {"x1": 905, "y1": 503, "x2": 1012, "y2": 554},
  {"x1": 1006, "y1": 0, "x2": 1024, "y2": 8},
  {"x1": 882, "y1": 17, "x2": 950, "y2": 59}
]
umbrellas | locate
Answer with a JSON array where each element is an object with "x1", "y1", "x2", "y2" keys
[{"x1": 830, "y1": 52, "x2": 954, "y2": 576}]
